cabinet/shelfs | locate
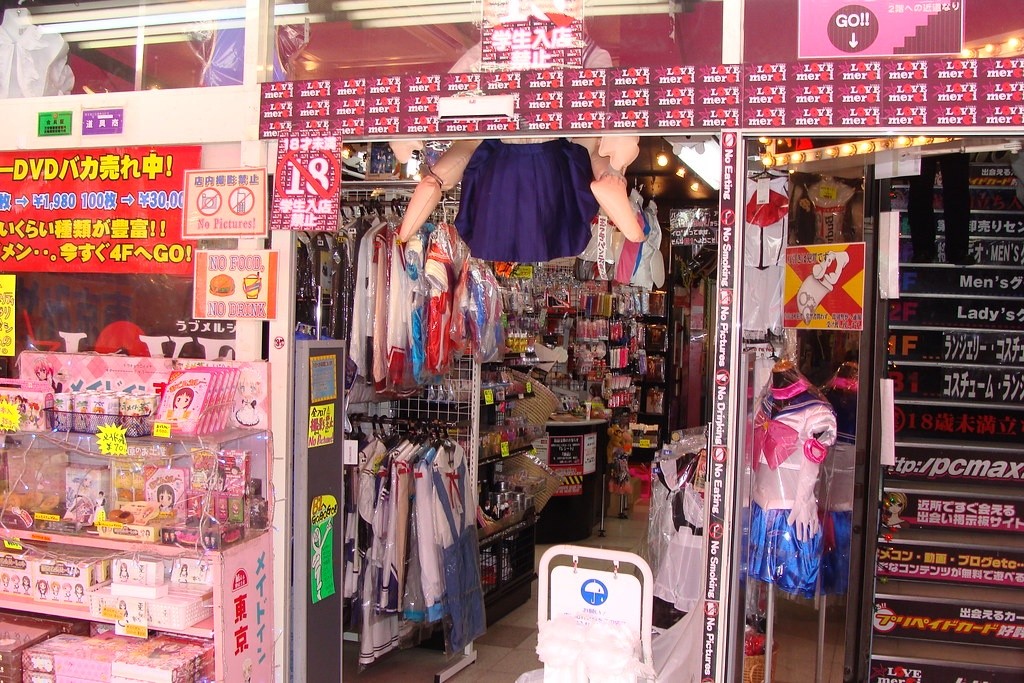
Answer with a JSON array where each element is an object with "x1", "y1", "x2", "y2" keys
[{"x1": 0, "y1": 525, "x2": 274, "y2": 683}]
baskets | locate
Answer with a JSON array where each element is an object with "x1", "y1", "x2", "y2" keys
[
  {"x1": 509, "y1": 366, "x2": 560, "y2": 435},
  {"x1": 504, "y1": 453, "x2": 563, "y2": 513}
]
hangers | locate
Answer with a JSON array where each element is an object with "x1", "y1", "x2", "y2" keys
[
  {"x1": 341, "y1": 198, "x2": 404, "y2": 229},
  {"x1": 343, "y1": 411, "x2": 465, "y2": 453}
]
